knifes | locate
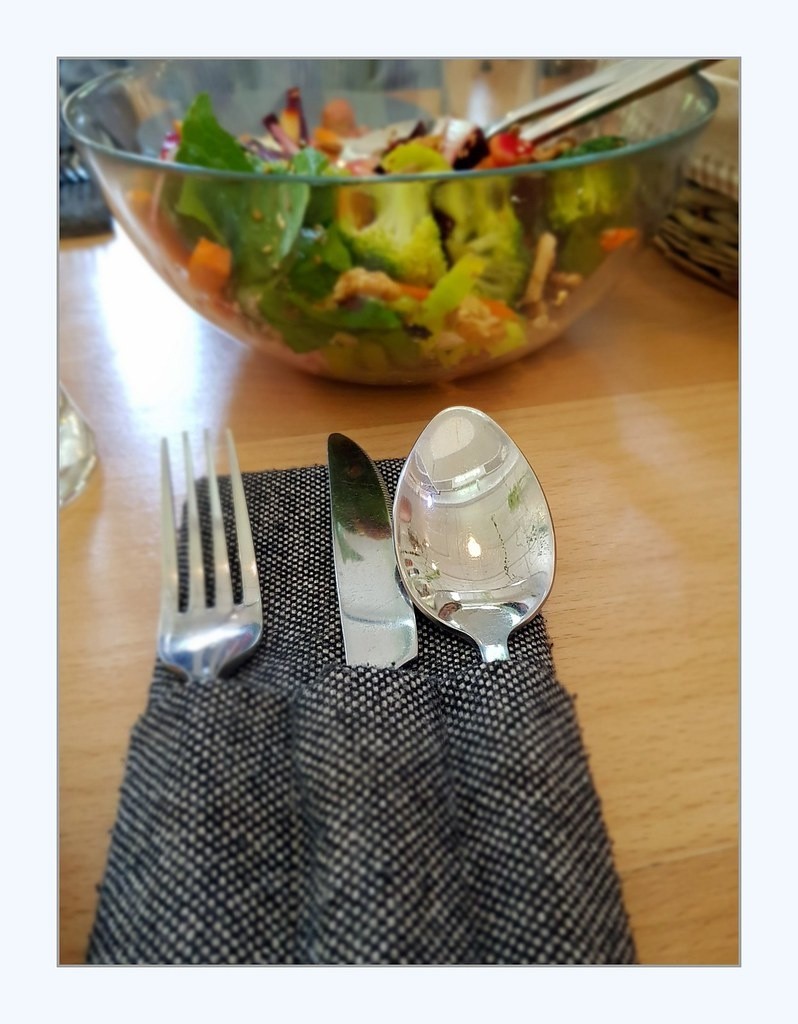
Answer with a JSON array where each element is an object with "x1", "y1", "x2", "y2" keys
[{"x1": 327, "y1": 433, "x2": 419, "y2": 671}]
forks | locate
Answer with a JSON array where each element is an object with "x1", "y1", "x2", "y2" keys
[{"x1": 157, "y1": 426, "x2": 264, "y2": 682}]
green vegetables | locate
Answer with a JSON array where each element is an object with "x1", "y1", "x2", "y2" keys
[{"x1": 157, "y1": 89, "x2": 427, "y2": 369}]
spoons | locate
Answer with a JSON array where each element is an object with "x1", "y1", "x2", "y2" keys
[{"x1": 392, "y1": 405, "x2": 556, "y2": 663}]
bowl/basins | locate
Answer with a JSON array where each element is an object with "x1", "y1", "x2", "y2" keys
[{"x1": 61, "y1": 59, "x2": 720, "y2": 387}]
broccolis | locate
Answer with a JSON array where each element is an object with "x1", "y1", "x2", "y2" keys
[{"x1": 336, "y1": 134, "x2": 635, "y2": 299}]
